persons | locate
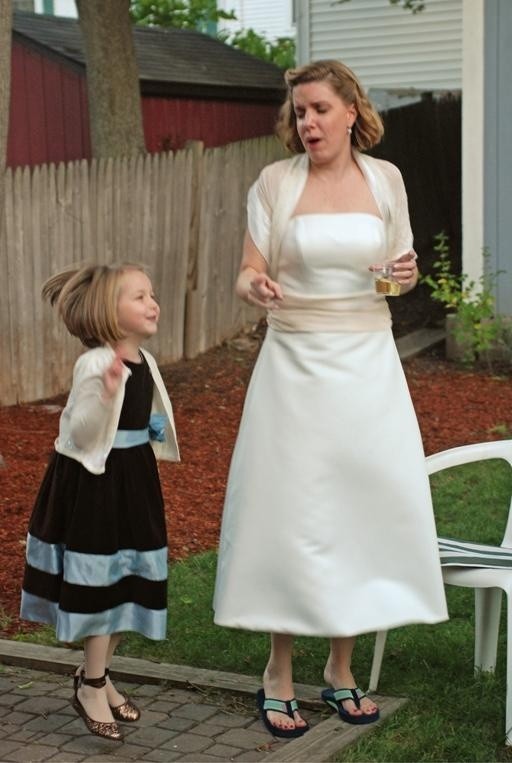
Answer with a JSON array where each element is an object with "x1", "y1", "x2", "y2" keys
[
  {"x1": 18, "y1": 260, "x2": 180, "y2": 741},
  {"x1": 212, "y1": 59, "x2": 451, "y2": 740}
]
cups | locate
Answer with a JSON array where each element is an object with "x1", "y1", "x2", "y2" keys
[{"x1": 374, "y1": 264, "x2": 401, "y2": 297}]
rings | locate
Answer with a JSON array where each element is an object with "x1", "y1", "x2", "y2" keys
[{"x1": 409, "y1": 269, "x2": 413, "y2": 279}]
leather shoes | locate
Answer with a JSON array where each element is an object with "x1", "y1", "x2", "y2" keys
[{"x1": 71, "y1": 667, "x2": 141, "y2": 740}]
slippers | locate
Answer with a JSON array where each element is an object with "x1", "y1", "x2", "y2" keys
[
  {"x1": 321, "y1": 688, "x2": 379, "y2": 724},
  {"x1": 256, "y1": 689, "x2": 309, "y2": 738}
]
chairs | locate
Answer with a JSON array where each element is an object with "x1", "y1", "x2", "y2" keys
[{"x1": 360, "y1": 438, "x2": 512, "y2": 749}]
